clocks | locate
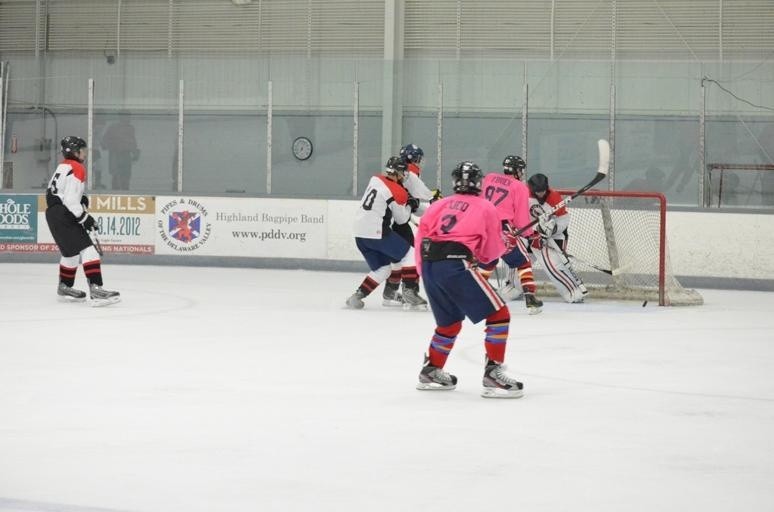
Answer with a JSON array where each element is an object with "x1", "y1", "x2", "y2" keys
[{"x1": 291, "y1": 137, "x2": 313, "y2": 161}]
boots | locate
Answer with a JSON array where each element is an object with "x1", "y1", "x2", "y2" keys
[
  {"x1": 345, "y1": 287, "x2": 367, "y2": 310},
  {"x1": 481, "y1": 353, "x2": 524, "y2": 391},
  {"x1": 381, "y1": 280, "x2": 403, "y2": 303},
  {"x1": 399, "y1": 281, "x2": 429, "y2": 306},
  {"x1": 524, "y1": 287, "x2": 544, "y2": 308},
  {"x1": 417, "y1": 351, "x2": 460, "y2": 387},
  {"x1": 86, "y1": 278, "x2": 120, "y2": 301}
]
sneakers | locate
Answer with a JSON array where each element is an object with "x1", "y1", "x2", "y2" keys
[{"x1": 56, "y1": 276, "x2": 86, "y2": 298}]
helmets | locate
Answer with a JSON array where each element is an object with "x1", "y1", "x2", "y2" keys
[
  {"x1": 397, "y1": 142, "x2": 424, "y2": 164},
  {"x1": 526, "y1": 173, "x2": 550, "y2": 194},
  {"x1": 449, "y1": 160, "x2": 487, "y2": 197},
  {"x1": 58, "y1": 134, "x2": 88, "y2": 155},
  {"x1": 384, "y1": 155, "x2": 409, "y2": 177},
  {"x1": 502, "y1": 154, "x2": 527, "y2": 177}
]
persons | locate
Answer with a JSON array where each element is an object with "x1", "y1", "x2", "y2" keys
[
  {"x1": 346, "y1": 157, "x2": 426, "y2": 305},
  {"x1": 46, "y1": 137, "x2": 118, "y2": 301},
  {"x1": 382, "y1": 143, "x2": 445, "y2": 303},
  {"x1": 617, "y1": 170, "x2": 664, "y2": 203},
  {"x1": 497, "y1": 173, "x2": 588, "y2": 303},
  {"x1": 664, "y1": 121, "x2": 701, "y2": 192},
  {"x1": 478, "y1": 156, "x2": 543, "y2": 308},
  {"x1": 413, "y1": 160, "x2": 524, "y2": 391},
  {"x1": 103, "y1": 111, "x2": 136, "y2": 191}
]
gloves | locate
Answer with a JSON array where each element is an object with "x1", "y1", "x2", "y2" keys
[
  {"x1": 528, "y1": 229, "x2": 545, "y2": 251},
  {"x1": 405, "y1": 195, "x2": 421, "y2": 213},
  {"x1": 72, "y1": 210, "x2": 100, "y2": 232},
  {"x1": 79, "y1": 195, "x2": 90, "y2": 209},
  {"x1": 536, "y1": 215, "x2": 558, "y2": 239},
  {"x1": 502, "y1": 230, "x2": 518, "y2": 257}
]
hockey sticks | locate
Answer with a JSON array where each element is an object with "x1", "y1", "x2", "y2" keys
[
  {"x1": 515, "y1": 139, "x2": 609, "y2": 236},
  {"x1": 567, "y1": 253, "x2": 634, "y2": 275}
]
